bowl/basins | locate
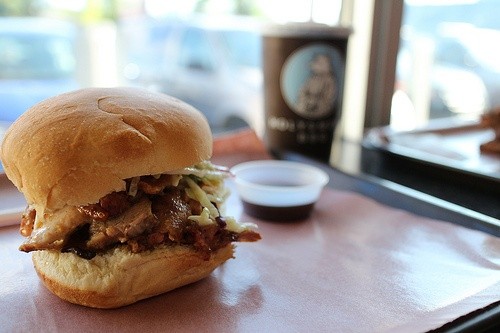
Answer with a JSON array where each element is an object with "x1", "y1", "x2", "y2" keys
[{"x1": 229, "y1": 159, "x2": 331, "y2": 223}]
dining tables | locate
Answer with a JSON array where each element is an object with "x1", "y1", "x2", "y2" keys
[{"x1": 1, "y1": 140, "x2": 500, "y2": 333}]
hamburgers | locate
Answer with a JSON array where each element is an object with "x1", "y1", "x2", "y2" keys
[{"x1": 0, "y1": 85, "x2": 262, "y2": 310}]
